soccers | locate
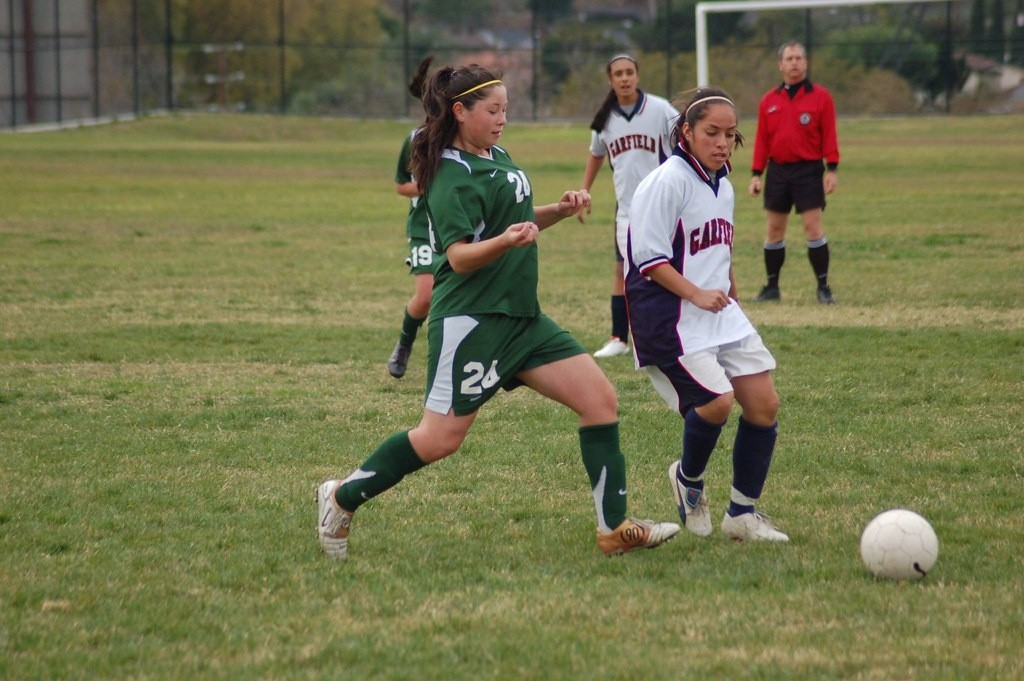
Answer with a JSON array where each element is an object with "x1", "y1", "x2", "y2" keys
[{"x1": 859, "y1": 508, "x2": 940, "y2": 581}]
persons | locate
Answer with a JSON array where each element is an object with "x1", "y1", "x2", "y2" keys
[
  {"x1": 748, "y1": 42, "x2": 839, "y2": 304},
  {"x1": 388, "y1": 54, "x2": 433, "y2": 379},
  {"x1": 315, "y1": 64, "x2": 682, "y2": 562},
  {"x1": 577, "y1": 53, "x2": 681, "y2": 357},
  {"x1": 623, "y1": 87, "x2": 789, "y2": 542}
]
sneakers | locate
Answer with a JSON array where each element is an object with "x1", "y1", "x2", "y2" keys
[
  {"x1": 722, "y1": 511, "x2": 789, "y2": 542},
  {"x1": 752, "y1": 285, "x2": 780, "y2": 301},
  {"x1": 817, "y1": 285, "x2": 832, "y2": 303},
  {"x1": 669, "y1": 460, "x2": 712, "y2": 536},
  {"x1": 314, "y1": 479, "x2": 354, "y2": 560},
  {"x1": 596, "y1": 517, "x2": 681, "y2": 558},
  {"x1": 594, "y1": 336, "x2": 629, "y2": 357},
  {"x1": 388, "y1": 341, "x2": 410, "y2": 378}
]
